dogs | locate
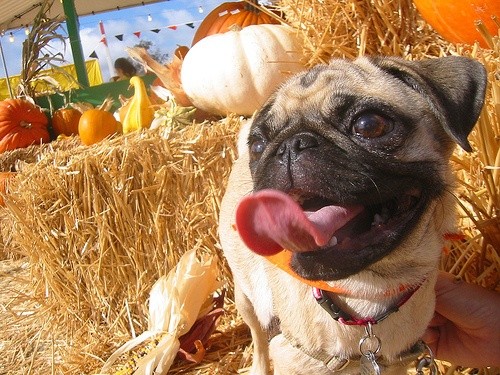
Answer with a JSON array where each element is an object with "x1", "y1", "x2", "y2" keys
[{"x1": 215, "y1": 53, "x2": 489, "y2": 374}]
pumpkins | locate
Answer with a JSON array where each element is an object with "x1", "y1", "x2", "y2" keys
[
  {"x1": 191, "y1": 0, "x2": 284, "y2": 45},
  {"x1": 78, "y1": 99, "x2": 117, "y2": 146},
  {"x1": 414, "y1": 0, "x2": 500, "y2": 49},
  {"x1": 53, "y1": 106, "x2": 81, "y2": 138},
  {"x1": 180, "y1": 23, "x2": 306, "y2": 117},
  {"x1": 1, "y1": 97, "x2": 50, "y2": 153},
  {"x1": 122, "y1": 75, "x2": 153, "y2": 134}
]
